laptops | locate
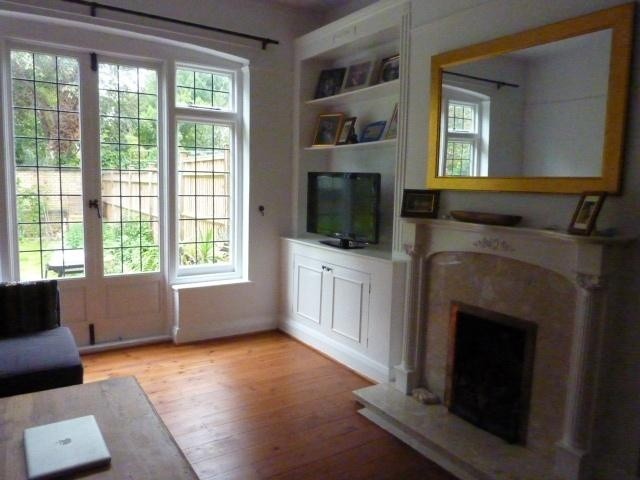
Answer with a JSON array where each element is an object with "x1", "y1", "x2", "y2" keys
[{"x1": 21, "y1": 414, "x2": 112, "y2": 479}]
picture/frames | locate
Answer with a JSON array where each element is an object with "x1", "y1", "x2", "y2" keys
[
  {"x1": 401, "y1": 189, "x2": 440, "y2": 218},
  {"x1": 311, "y1": 112, "x2": 344, "y2": 148},
  {"x1": 568, "y1": 191, "x2": 608, "y2": 235},
  {"x1": 360, "y1": 121, "x2": 387, "y2": 142},
  {"x1": 336, "y1": 117, "x2": 357, "y2": 144},
  {"x1": 340, "y1": 59, "x2": 376, "y2": 93},
  {"x1": 376, "y1": 54, "x2": 400, "y2": 84},
  {"x1": 384, "y1": 104, "x2": 398, "y2": 140},
  {"x1": 314, "y1": 67, "x2": 346, "y2": 100}
]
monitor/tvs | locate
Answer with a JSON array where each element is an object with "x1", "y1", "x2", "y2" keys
[{"x1": 306, "y1": 171, "x2": 382, "y2": 250}]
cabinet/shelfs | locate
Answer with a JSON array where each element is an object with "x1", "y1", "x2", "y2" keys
[{"x1": 279, "y1": 0, "x2": 406, "y2": 383}]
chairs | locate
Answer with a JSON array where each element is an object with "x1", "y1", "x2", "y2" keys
[{"x1": 0, "y1": 280, "x2": 83, "y2": 397}]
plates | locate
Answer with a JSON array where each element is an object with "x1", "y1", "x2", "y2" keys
[{"x1": 450, "y1": 209, "x2": 522, "y2": 227}]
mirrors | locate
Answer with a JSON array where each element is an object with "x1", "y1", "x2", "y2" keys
[{"x1": 425, "y1": 2, "x2": 637, "y2": 192}]
